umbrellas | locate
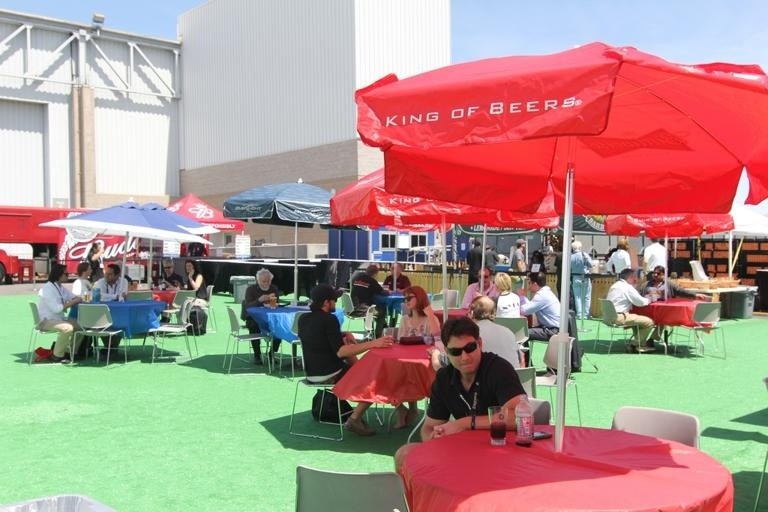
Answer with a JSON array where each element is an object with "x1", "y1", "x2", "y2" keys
[{"x1": 352, "y1": 41, "x2": 768, "y2": 452}]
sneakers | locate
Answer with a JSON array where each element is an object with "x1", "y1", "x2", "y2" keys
[{"x1": 344, "y1": 416, "x2": 377, "y2": 436}]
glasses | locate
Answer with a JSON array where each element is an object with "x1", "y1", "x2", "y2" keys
[
  {"x1": 445, "y1": 341, "x2": 480, "y2": 357},
  {"x1": 403, "y1": 295, "x2": 416, "y2": 302},
  {"x1": 326, "y1": 297, "x2": 337, "y2": 302}
]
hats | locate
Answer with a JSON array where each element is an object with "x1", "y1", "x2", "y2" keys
[{"x1": 310, "y1": 283, "x2": 344, "y2": 299}]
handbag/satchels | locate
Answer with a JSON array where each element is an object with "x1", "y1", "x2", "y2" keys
[{"x1": 311, "y1": 389, "x2": 355, "y2": 425}]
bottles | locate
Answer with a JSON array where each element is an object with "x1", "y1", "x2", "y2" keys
[
  {"x1": 162, "y1": 278, "x2": 166, "y2": 291},
  {"x1": 514, "y1": 394, "x2": 533, "y2": 446},
  {"x1": 433, "y1": 335, "x2": 444, "y2": 353}
]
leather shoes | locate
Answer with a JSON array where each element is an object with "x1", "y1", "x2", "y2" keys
[{"x1": 634, "y1": 344, "x2": 657, "y2": 355}]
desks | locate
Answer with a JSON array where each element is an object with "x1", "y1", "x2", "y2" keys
[
  {"x1": 375, "y1": 293, "x2": 432, "y2": 325},
  {"x1": 134, "y1": 288, "x2": 180, "y2": 310},
  {"x1": 435, "y1": 307, "x2": 470, "y2": 327},
  {"x1": 331, "y1": 341, "x2": 443, "y2": 435},
  {"x1": 391, "y1": 426, "x2": 736, "y2": 512},
  {"x1": 246, "y1": 305, "x2": 345, "y2": 370},
  {"x1": 629, "y1": 298, "x2": 712, "y2": 355},
  {"x1": 68, "y1": 297, "x2": 168, "y2": 355}
]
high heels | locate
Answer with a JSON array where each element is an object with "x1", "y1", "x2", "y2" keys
[{"x1": 394, "y1": 411, "x2": 419, "y2": 429}]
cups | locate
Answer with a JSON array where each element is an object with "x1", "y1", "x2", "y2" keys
[
  {"x1": 128, "y1": 282, "x2": 132, "y2": 291},
  {"x1": 424, "y1": 337, "x2": 432, "y2": 344},
  {"x1": 384, "y1": 327, "x2": 394, "y2": 338},
  {"x1": 84, "y1": 292, "x2": 90, "y2": 304},
  {"x1": 488, "y1": 405, "x2": 509, "y2": 446},
  {"x1": 133, "y1": 280, "x2": 137, "y2": 290},
  {"x1": 270, "y1": 297, "x2": 277, "y2": 310}
]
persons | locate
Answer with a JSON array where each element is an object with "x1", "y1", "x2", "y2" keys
[
  {"x1": 36, "y1": 243, "x2": 209, "y2": 363},
  {"x1": 240, "y1": 237, "x2": 708, "y2": 441}
]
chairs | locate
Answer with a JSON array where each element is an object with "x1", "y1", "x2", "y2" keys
[
  {"x1": 24, "y1": 302, "x2": 69, "y2": 364},
  {"x1": 163, "y1": 290, "x2": 198, "y2": 323},
  {"x1": 374, "y1": 326, "x2": 403, "y2": 426},
  {"x1": 127, "y1": 291, "x2": 154, "y2": 300},
  {"x1": 611, "y1": 404, "x2": 702, "y2": 451},
  {"x1": 431, "y1": 295, "x2": 446, "y2": 309},
  {"x1": 150, "y1": 296, "x2": 198, "y2": 364},
  {"x1": 69, "y1": 304, "x2": 128, "y2": 366},
  {"x1": 495, "y1": 316, "x2": 530, "y2": 345},
  {"x1": 528, "y1": 338, "x2": 557, "y2": 375},
  {"x1": 441, "y1": 289, "x2": 459, "y2": 306},
  {"x1": 594, "y1": 297, "x2": 644, "y2": 355},
  {"x1": 341, "y1": 317, "x2": 375, "y2": 332},
  {"x1": 521, "y1": 398, "x2": 551, "y2": 424},
  {"x1": 515, "y1": 365, "x2": 536, "y2": 396},
  {"x1": 270, "y1": 335, "x2": 292, "y2": 373},
  {"x1": 223, "y1": 306, "x2": 273, "y2": 375},
  {"x1": 691, "y1": 302, "x2": 728, "y2": 359},
  {"x1": 288, "y1": 344, "x2": 369, "y2": 441},
  {"x1": 188, "y1": 283, "x2": 216, "y2": 334},
  {"x1": 342, "y1": 292, "x2": 355, "y2": 315},
  {"x1": 535, "y1": 334, "x2": 583, "y2": 427}
]
